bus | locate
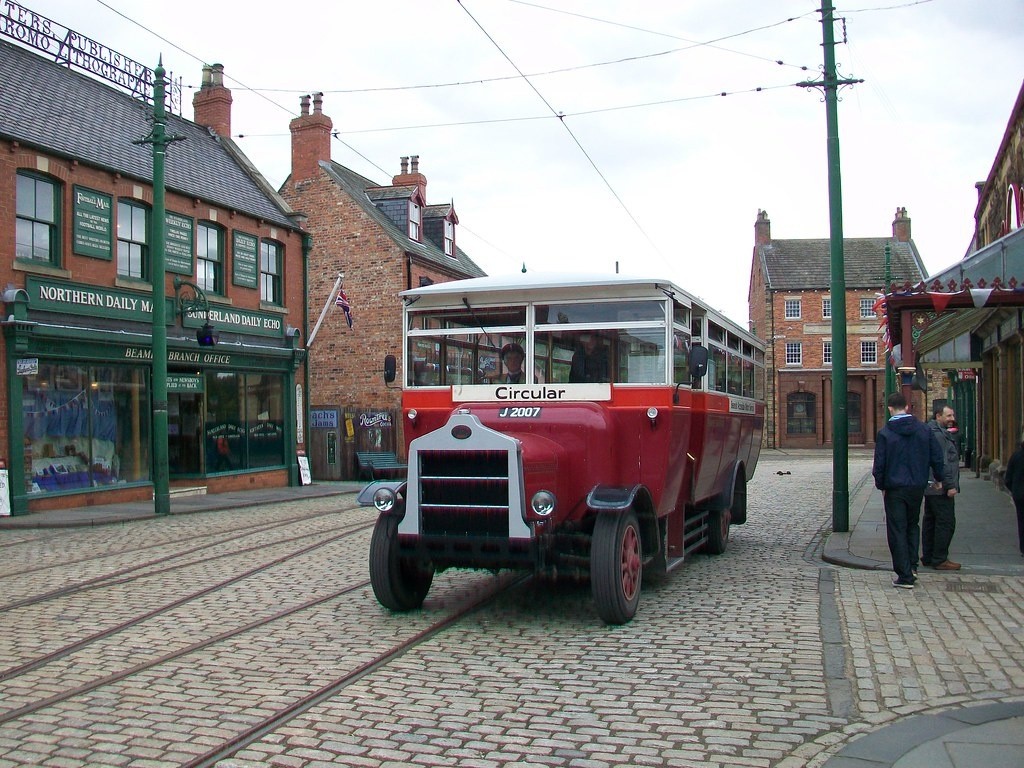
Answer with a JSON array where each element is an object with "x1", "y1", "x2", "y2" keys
[{"x1": 357, "y1": 263, "x2": 768, "y2": 624}]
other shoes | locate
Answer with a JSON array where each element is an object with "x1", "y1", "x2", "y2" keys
[
  {"x1": 912, "y1": 566, "x2": 919, "y2": 579},
  {"x1": 892, "y1": 578, "x2": 914, "y2": 588},
  {"x1": 936, "y1": 560, "x2": 961, "y2": 570},
  {"x1": 923, "y1": 563, "x2": 936, "y2": 566}
]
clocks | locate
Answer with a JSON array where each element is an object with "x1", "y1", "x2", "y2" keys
[{"x1": 792, "y1": 399, "x2": 807, "y2": 432}]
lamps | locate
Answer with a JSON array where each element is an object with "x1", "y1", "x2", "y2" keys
[{"x1": 173, "y1": 275, "x2": 219, "y2": 346}]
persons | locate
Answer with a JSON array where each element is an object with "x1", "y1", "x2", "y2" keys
[
  {"x1": 1003, "y1": 433, "x2": 1024, "y2": 556},
  {"x1": 926, "y1": 403, "x2": 962, "y2": 570},
  {"x1": 568, "y1": 330, "x2": 610, "y2": 383},
  {"x1": 491, "y1": 343, "x2": 538, "y2": 384},
  {"x1": 871, "y1": 393, "x2": 945, "y2": 589}
]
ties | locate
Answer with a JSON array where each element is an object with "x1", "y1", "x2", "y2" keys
[{"x1": 507, "y1": 370, "x2": 522, "y2": 383}]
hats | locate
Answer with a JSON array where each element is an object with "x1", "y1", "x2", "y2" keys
[{"x1": 501, "y1": 343, "x2": 525, "y2": 361}]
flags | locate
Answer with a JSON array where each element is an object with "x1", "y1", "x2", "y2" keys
[{"x1": 334, "y1": 284, "x2": 354, "y2": 331}]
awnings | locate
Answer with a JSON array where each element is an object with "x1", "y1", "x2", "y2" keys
[{"x1": 875, "y1": 230, "x2": 1023, "y2": 359}]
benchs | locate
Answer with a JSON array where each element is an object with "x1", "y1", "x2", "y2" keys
[{"x1": 356, "y1": 452, "x2": 408, "y2": 481}]
